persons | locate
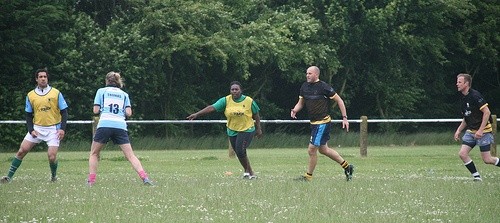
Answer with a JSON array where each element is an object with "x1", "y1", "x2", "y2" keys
[
  {"x1": 454, "y1": 74, "x2": 500, "y2": 180},
  {"x1": 0, "y1": 69, "x2": 68, "y2": 182},
  {"x1": 186, "y1": 81, "x2": 262, "y2": 178},
  {"x1": 291, "y1": 66, "x2": 353, "y2": 182},
  {"x1": 87, "y1": 72, "x2": 154, "y2": 187}
]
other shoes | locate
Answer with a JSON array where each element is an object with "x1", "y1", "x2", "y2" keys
[
  {"x1": 143, "y1": 178, "x2": 154, "y2": 185},
  {"x1": 473, "y1": 179, "x2": 482, "y2": 182},
  {"x1": 51, "y1": 176, "x2": 57, "y2": 181},
  {"x1": 2, "y1": 175, "x2": 10, "y2": 181}
]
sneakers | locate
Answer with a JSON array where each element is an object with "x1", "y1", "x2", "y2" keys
[
  {"x1": 295, "y1": 176, "x2": 311, "y2": 182},
  {"x1": 345, "y1": 165, "x2": 354, "y2": 181}
]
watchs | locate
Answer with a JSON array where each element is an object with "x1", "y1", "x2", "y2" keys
[{"x1": 343, "y1": 116, "x2": 347, "y2": 118}]
